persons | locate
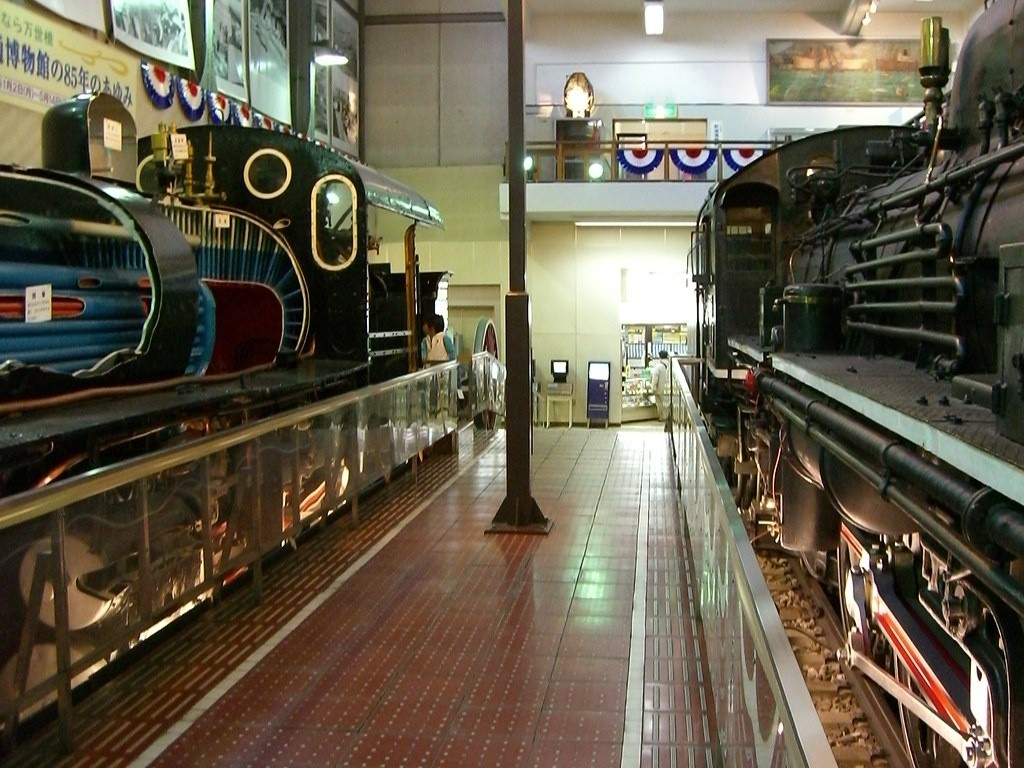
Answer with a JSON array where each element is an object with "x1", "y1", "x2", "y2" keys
[
  {"x1": 652, "y1": 350, "x2": 670, "y2": 422},
  {"x1": 420, "y1": 313, "x2": 456, "y2": 368}
]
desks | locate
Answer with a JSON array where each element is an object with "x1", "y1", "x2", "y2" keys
[{"x1": 546, "y1": 395, "x2": 574, "y2": 429}]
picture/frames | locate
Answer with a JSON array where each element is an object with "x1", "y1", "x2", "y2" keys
[{"x1": 765, "y1": 38, "x2": 927, "y2": 107}]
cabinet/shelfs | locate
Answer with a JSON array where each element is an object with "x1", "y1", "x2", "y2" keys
[{"x1": 622, "y1": 378, "x2": 659, "y2": 423}]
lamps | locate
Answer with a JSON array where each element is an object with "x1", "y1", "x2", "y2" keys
[
  {"x1": 310, "y1": 40, "x2": 349, "y2": 67},
  {"x1": 643, "y1": 0, "x2": 665, "y2": 35}
]
